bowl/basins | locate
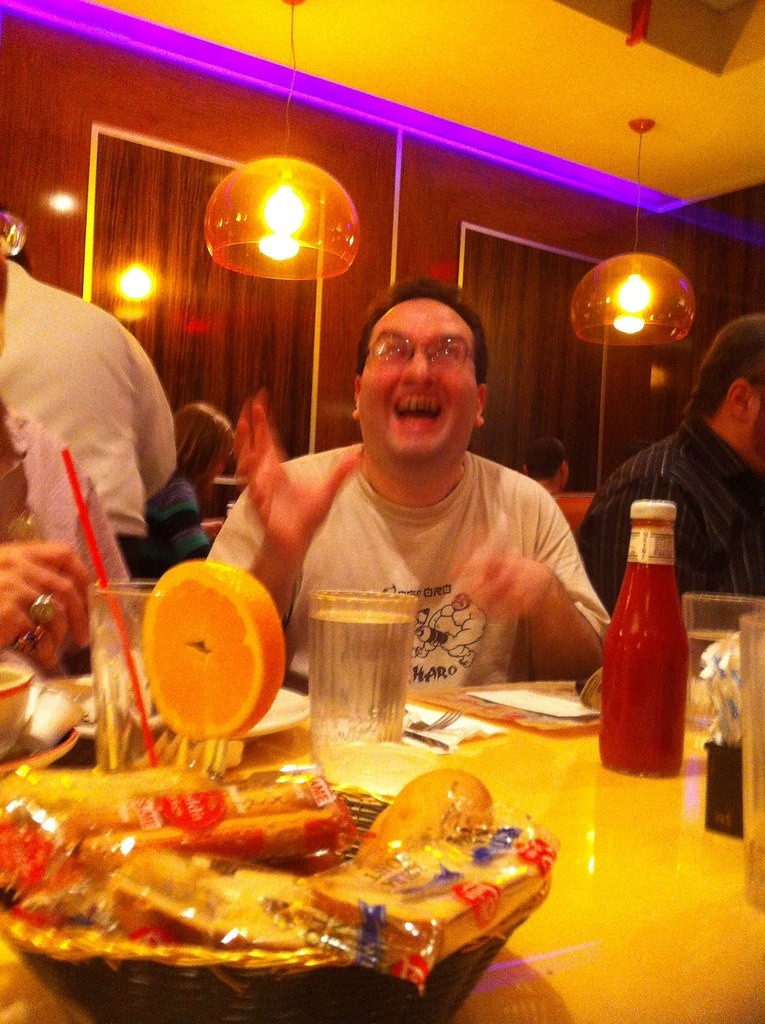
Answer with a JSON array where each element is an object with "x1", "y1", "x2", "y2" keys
[{"x1": 0, "y1": 660, "x2": 39, "y2": 759}]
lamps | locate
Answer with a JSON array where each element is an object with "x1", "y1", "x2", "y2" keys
[
  {"x1": 205, "y1": 0, "x2": 361, "y2": 284},
  {"x1": 572, "y1": 118, "x2": 697, "y2": 346}
]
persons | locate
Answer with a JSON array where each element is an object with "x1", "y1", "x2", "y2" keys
[
  {"x1": 576, "y1": 312, "x2": 765, "y2": 618},
  {"x1": 115, "y1": 399, "x2": 234, "y2": 593},
  {"x1": 206, "y1": 275, "x2": 612, "y2": 697},
  {"x1": 0, "y1": 202, "x2": 213, "y2": 583},
  {"x1": 0, "y1": 391, "x2": 134, "y2": 672},
  {"x1": 522, "y1": 436, "x2": 569, "y2": 495}
]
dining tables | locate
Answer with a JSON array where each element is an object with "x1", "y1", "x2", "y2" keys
[{"x1": 0, "y1": 680, "x2": 765, "y2": 1024}]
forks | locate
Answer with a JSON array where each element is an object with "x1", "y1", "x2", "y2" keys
[{"x1": 403, "y1": 706, "x2": 468, "y2": 735}]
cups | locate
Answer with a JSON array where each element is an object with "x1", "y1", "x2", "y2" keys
[
  {"x1": 307, "y1": 590, "x2": 419, "y2": 788},
  {"x1": 680, "y1": 591, "x2": 765, "y2": 751},
  {"x1": 736, "y1": 612, "x2": 765, "y2": 920},
  {"x1": 84, "y1": 579, "x2": 233, "y2": 790}
]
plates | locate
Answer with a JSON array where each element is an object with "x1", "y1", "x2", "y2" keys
[
  {"x1": 41, "y1": 670, "x2": 311, "y2": 742},
  {"x1": 0, "y1": 720, "x2": 83, "y2": 779}
]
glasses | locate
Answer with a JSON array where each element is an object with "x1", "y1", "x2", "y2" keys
[{"x1": 364, "y1": 332, "x2": 475, "y2": 371}]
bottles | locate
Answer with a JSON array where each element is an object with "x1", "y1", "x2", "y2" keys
[{"x1": 599, "y1": 499, "x2": 690, "y2": 780}]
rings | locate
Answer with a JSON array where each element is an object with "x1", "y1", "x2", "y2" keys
[
  {"x1": 29, "y1": 593, "x2": 55, "y2": 623},
  {"x1": 11, "y1": 624, "x2": 46, "y2": 659}
]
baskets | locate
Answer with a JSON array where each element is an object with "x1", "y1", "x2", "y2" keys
[{"x1": 0, "y1": 786, "x2": 554, "y2": 1022}]
